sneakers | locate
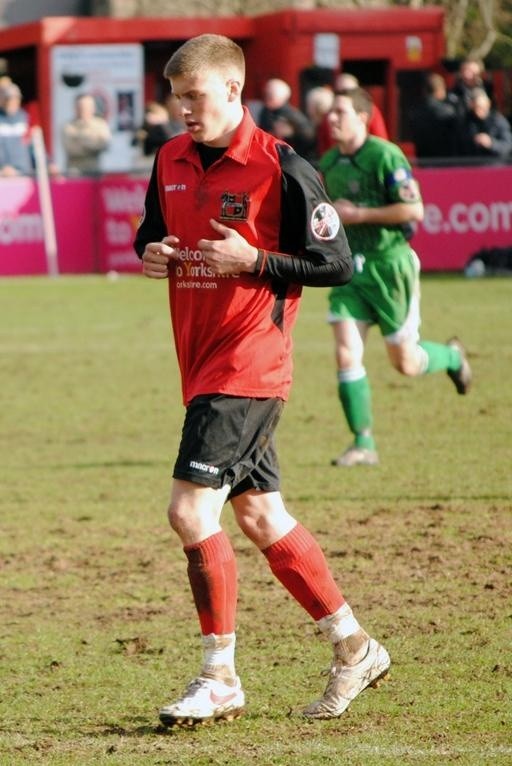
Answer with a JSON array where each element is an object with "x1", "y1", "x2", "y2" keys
[
  {"x1": 304, "y1": 639, "x2": 390, "y2": 719},
  {"x1": 160, "y1": 678, "x2": 245, "y2": 722},
  {"x1": 448, "y1": 337, "x2": 471, "y2": 394},
  {"x1": 331, "y1": 447, "x2": 378, "y2": 466}
]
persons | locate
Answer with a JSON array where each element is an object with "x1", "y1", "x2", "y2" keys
[
  {"x1": 314, "y1": 90, "x2": 474, "y2": 465},
  {"x1": 0, "y1": 48, "x2": 511, "y2": 180},
  {"x1": 132, "y1": 32, "x2": 392, "y2": 726}
]
